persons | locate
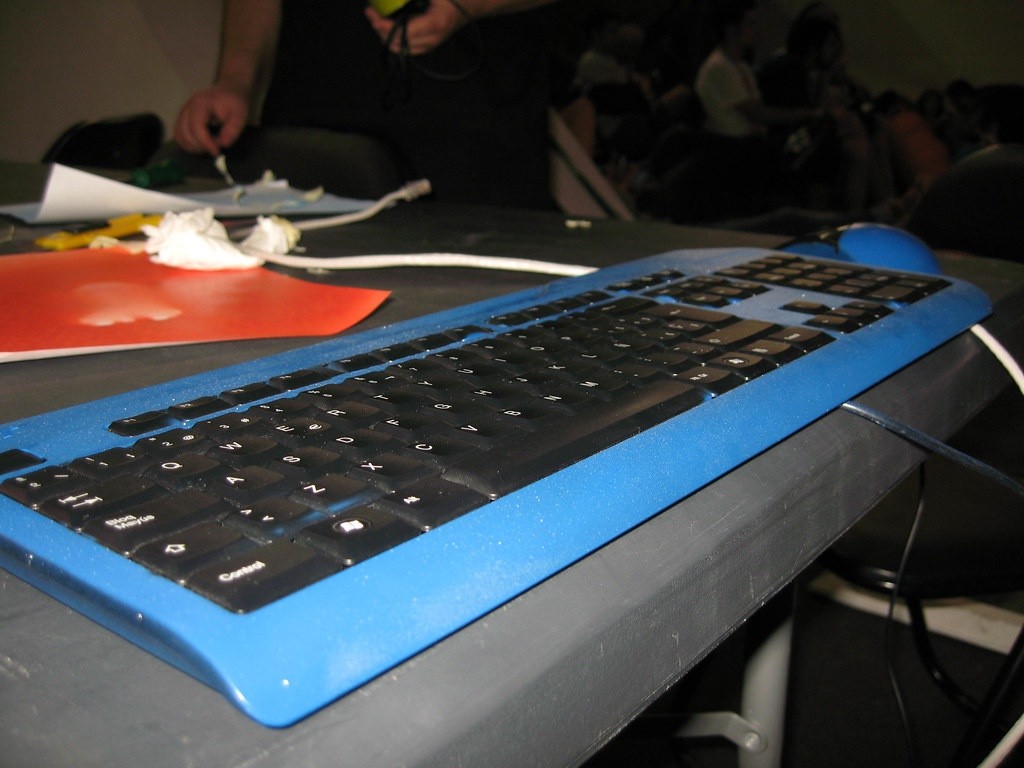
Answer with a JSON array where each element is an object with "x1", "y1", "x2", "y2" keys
[
  {"x1": 172, "y1": 0, "x2": 554, "y2": 213},
  {"x1": 571, "y1": 8, "x2": 1024, "y2": 265}
]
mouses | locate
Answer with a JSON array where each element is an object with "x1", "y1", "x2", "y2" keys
[{"x1": 778, "y1": 221, "x2": 938, "y2": 276}]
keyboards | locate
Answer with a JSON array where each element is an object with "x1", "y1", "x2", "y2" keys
[{"x1": 0, "y1": 244, "x2": 994, "y2": 725}]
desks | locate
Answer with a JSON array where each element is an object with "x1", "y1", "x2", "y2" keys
[{"x1": 1, "y1": 172, "x2": 1024, "y2": 768}]
